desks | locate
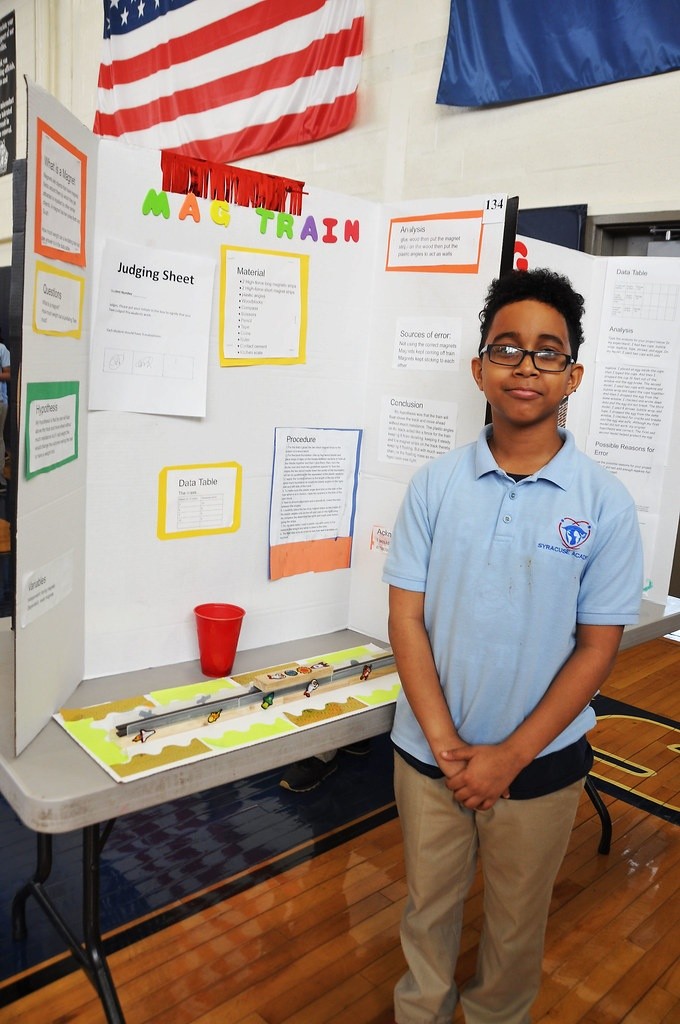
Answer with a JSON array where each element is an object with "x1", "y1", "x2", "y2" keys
[{"x1": 0, "y1": 589, "x2": 679, "y2": 1024}]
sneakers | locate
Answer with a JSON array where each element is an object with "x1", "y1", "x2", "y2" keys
[
  {"x1": 279, "y1": 758, "x2": 337, "y2": 792},
  {"x1": 343, "y1": 741, "x2": 369, "y2": 755}
]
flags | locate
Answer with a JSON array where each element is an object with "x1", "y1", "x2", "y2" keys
[
  {"x1": 91, "y1": 0, "x2": 365, "y2": 164},
  {"x1": 435, "y1": 0, "x2": 679, "y2": 111}
]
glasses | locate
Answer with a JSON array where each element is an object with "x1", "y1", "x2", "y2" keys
[{"x1": 480, "y1": 344, "x2": 574, "y2": 372}]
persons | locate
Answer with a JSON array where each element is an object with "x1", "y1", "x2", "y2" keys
[{"x1": 390, "y1": 268, "x2": 645, "y2": 1024}]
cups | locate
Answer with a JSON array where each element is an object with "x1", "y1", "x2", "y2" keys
[{"x1": 194, "y1": 603, "x2": 246, "y2": 677}]
